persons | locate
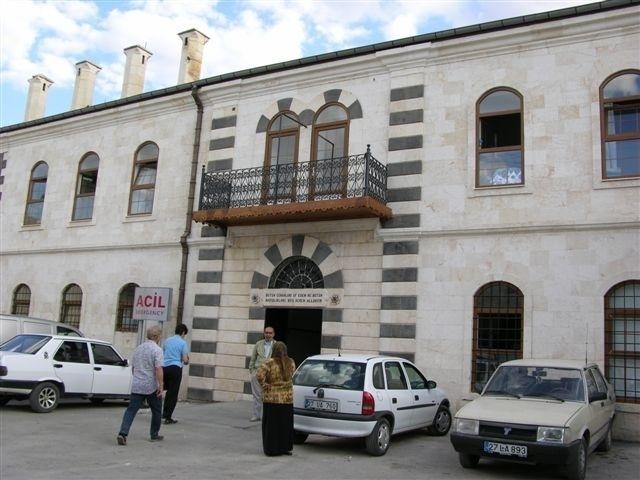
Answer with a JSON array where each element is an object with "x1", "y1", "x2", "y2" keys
[
  {"x1": 163, "y1": 324, "x2": 189, "y2": 424},
  {"x1": 249, "y1": 327, "x2": 277, "y2": 422},
  {"x1": 117, "y1": 325, "x2": 165, "y2": 445},
  {"x1": 256, "y1": 341, "x2": 294, "y2": 457}
]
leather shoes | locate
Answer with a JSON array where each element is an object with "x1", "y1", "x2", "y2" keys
[{"x1": 164, "y1": 419, "x2": 178, "y2": 424}]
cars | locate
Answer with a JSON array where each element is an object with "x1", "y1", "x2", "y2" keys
[
  {"x1": 448, "y1": 358, "x2": 616, "y2": 479},
  {"x1": 0, "y1": 334, "x2": 133, "y2": 413},
  {"x1": 289, "y1": 353, "x2": 452, "y2": 455}
]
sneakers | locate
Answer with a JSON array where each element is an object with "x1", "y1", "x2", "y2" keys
[
  {"x1": 116, "y1": 435, "x2": 127, "y2": 445},
  {"x1": 250, "y1": 417, "x2": 260, "y2": 422},
  {"x1": 150, "y1": 436, "x2": 164, "y2": 442}
]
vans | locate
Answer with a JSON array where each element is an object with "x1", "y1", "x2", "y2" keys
[{"x1": 0, "y1": 314, "x2": 84, "y2": 344}]
